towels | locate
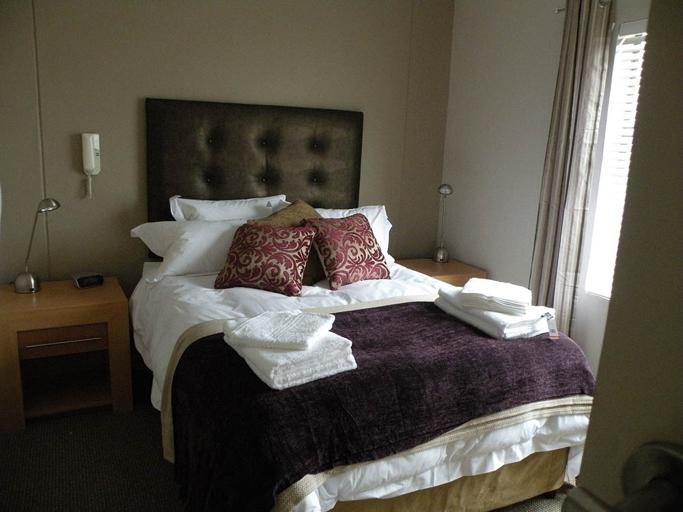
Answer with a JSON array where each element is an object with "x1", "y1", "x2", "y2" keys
[
  {"x1": 433, "y1": 278, "x2": 555, "y2": 340},
  {"x1": 219, "y1": 309, "x2": 357, "y2": 392}
]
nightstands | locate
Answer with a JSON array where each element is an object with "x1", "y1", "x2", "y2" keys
[
  {"x1": 395, "y1": 255, "x2": 488, "y2": 288},
  {"x1": 1, "y1": 277, "x2": 133, "y2": 433}
]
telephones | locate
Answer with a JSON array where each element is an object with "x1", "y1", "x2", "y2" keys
[{"x1": 82, "y1": 133, "x2": 101, "y2": 175}]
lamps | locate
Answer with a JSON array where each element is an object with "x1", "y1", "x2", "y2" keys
[
  {"x1": 12, "y1": 197, "x2": 61, "y2": 294},
  {"x1": 433, "y1": 183, "x2": 452, "y2": 263}
]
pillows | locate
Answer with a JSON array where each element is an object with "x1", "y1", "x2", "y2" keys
[{"x1": 129, "y1": 194, "x2": 392, "y2": 296}]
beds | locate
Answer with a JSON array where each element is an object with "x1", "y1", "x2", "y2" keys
[{"x1": 121, "y1": 96, "x2": 597, "y2": 512}]
